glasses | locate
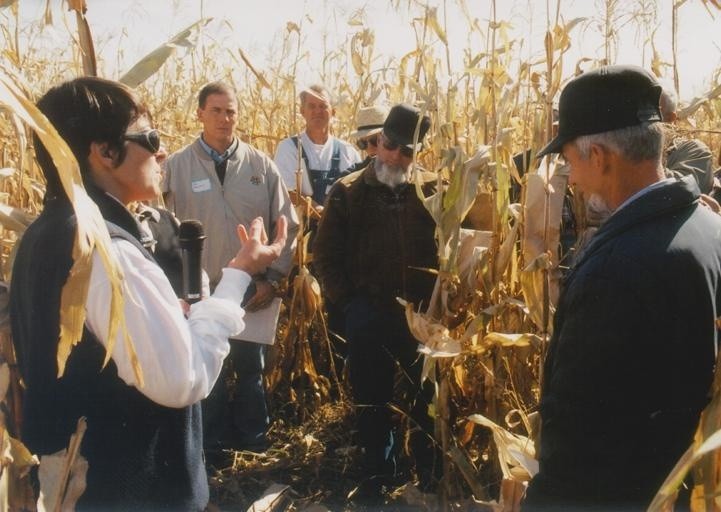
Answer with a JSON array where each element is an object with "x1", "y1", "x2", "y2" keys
[
  {"x1": 383, "y1": 137, "x2": 413, "y2": 157},
  {"x1": 357, "y1": 136, "x2": 378, "y2": 150},
  {"x1": 125, "y1": 129, "x2": 160, "y2": 153}
]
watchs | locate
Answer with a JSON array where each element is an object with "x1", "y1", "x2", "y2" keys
[{"x1": 267, "y1": 278, "x2": 280, "y2": 289}]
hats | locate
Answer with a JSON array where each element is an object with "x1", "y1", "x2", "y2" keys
[
  {"x1": 535, "y1": 64, "x2": 662, "y2": 158},
  {"x1": 350, "y1": 104, "x2": 431, "y2": 144}
]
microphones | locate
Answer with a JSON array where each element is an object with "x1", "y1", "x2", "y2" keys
[{"x1": 178, "y1": 219, "x2": 207, "y2": 304}]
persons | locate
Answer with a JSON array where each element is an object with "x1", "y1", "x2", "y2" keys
[
  {"x1": 275, "y1": 85, "x2": 363, "y2": 320},
  {"x1": 10, "y1": 76, "x2": 286, "y2": 512},
  {"x1": 152, "y1": 79, "x2": 300, "y2": 466},
  {"x1": 520, "y1": 67, "x2": 721, "y2": 512}
]
